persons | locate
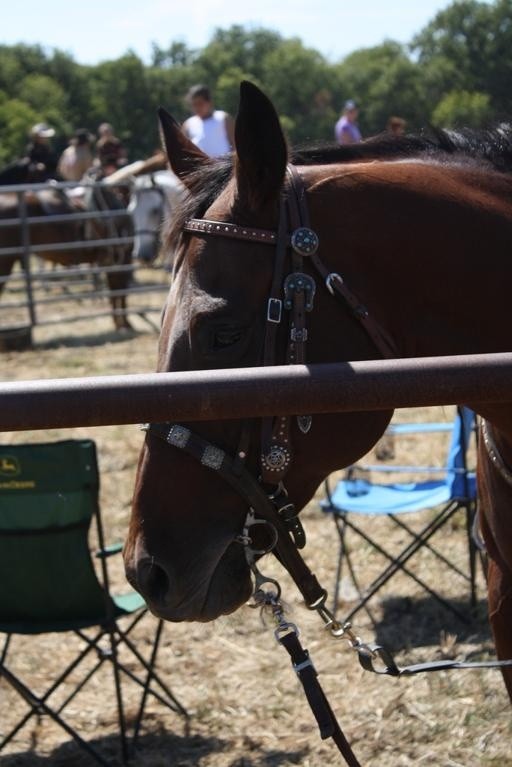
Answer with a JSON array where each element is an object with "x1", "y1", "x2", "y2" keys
[
  {"x1": 95, "y1": 152, "x2": 121, "y2": 195},
  {"x1": 13, "y1": 121, "x2": 58, "y2": 182},
  {"x1": 333, "y1": 97, "x2": 362, "y2": 145},
  {"x1": 94, "y1": 122, "x2": 127, "y2": 177},
  {"x1": 142, "y1": 84, "x2": 236, "y2": 173},
  {"x1": 55, "y1": 128, "x2": 95, "y2": 186},
  {"x1": 389, "y1": 116, "x2": 408, "y2": 136}
]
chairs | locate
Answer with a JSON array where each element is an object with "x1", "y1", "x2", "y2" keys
[
  {"x1": 1, "y1": 439, "x2": 190, "y2": 767},
  {"x1": 319, "y1": 401, "x2": 484, "y2": 629}
]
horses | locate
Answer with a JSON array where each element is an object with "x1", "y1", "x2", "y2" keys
[
  {"x1": 119, "y1": 79, "x2": 510, "y2": 705},
  {"x1": 0, "y1": 151, "x2": 207, "y2": 335}
]
